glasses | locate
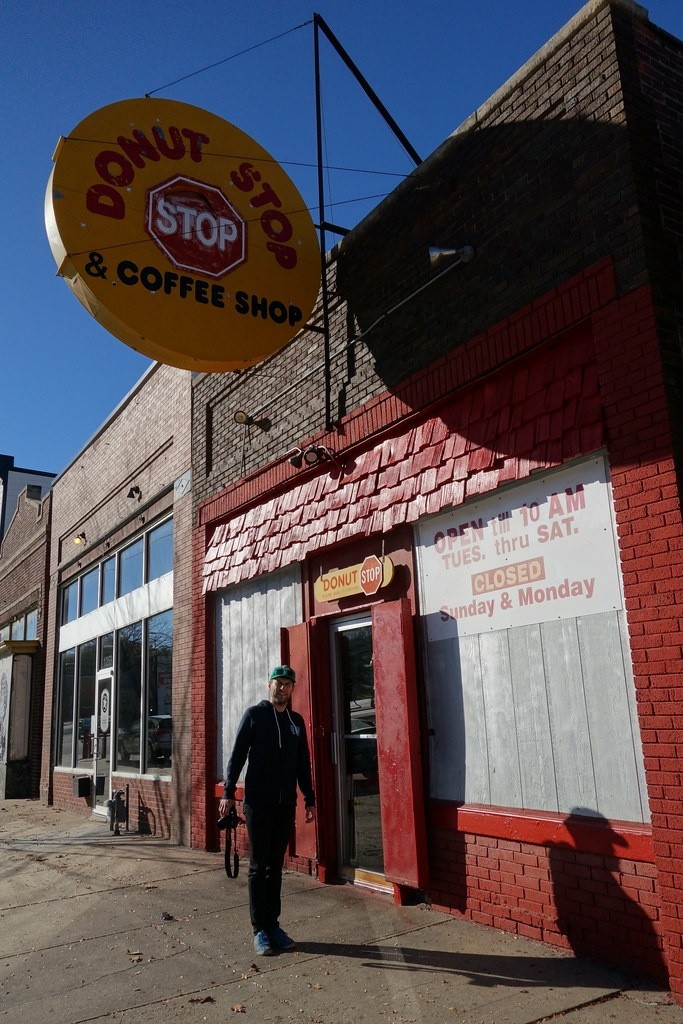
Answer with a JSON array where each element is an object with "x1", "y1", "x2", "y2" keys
[{"x1": 273, "y1": 669, "x2": 295, "y2": 677}]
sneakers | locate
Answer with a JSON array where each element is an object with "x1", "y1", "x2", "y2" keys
[
  {"x1": 271, "y1": 928, "x2": 296, "y2": 949},
  {"x1": 253, "y1": 931, "x2": 274, "y2": 957}
]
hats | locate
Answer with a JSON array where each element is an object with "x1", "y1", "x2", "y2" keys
[{"x1": 271, "y1": 665, "x2": 296, "y2": 683}]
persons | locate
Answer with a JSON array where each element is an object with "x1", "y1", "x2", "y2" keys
[{"x1": 218, "y1": 665, "x2": 315, "y2": 956}]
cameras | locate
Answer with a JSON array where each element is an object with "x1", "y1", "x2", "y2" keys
[{"x1": 217, "y1": 807, "x2": 238, "y2": 828}]
